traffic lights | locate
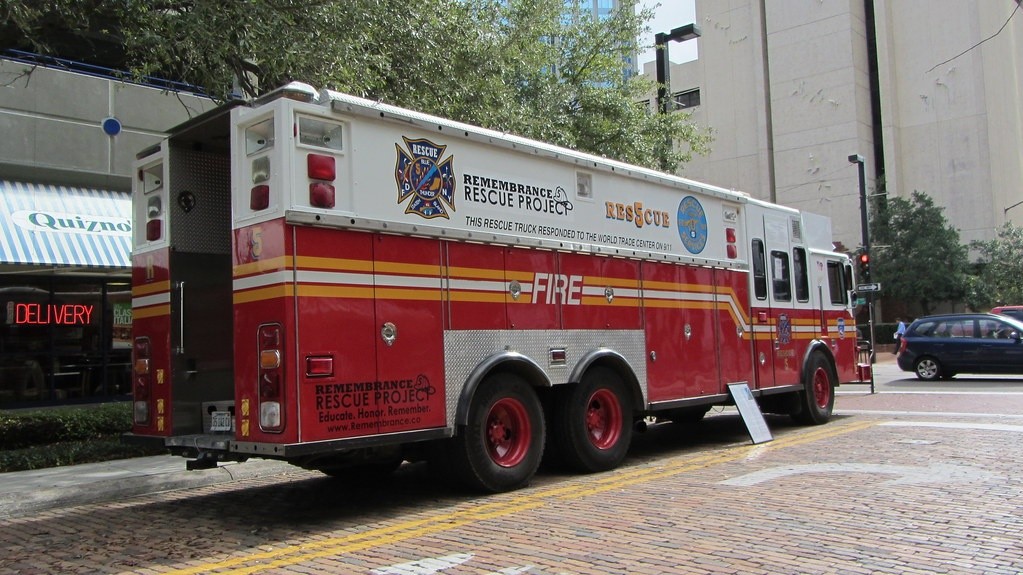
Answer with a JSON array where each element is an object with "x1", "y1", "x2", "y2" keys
[{"x1": 860, "y1": 252, "x2": 870, "y2": 279}]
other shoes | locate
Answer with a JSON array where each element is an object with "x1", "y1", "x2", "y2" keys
[{"x1": 892, "y1": 352, "x2": 897, "y2": 355}]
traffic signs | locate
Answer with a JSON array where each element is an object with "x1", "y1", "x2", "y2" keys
[{"x1": 858, "y1": 283, "x2": 881, "y2": 292}]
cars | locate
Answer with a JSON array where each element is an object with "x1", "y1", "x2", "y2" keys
[{"x1": 940, "y1": 306, "x2": 1023, "y2": 336}]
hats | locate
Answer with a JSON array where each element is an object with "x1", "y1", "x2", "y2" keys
[{"x1": 895, "y1": 317, "x2": 901, "y2": 320}]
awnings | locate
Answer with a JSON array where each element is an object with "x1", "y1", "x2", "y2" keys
[{"x1": 0, "y1": 178, "x2": 134, "y2": 270}]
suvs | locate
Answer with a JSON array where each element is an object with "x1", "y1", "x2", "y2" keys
[{"x1": 897, "y1": 313, "x2": 1023, "y2": 380}]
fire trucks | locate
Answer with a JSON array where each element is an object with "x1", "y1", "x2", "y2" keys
[{"x1": 118, "y1": 80, "x2": 862, "y2": 494}]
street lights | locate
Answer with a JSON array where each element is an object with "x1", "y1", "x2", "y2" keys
[
  {"x1": 653, "y1": 21, "x2": 703, "y2": 173},
  {"x1": 848, "y1": 154, "x2": 877, "y2": 363}
]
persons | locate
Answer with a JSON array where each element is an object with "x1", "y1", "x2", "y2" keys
[{"x1": 891, "y1": 318, "x2": 905, "y2": 354}]
